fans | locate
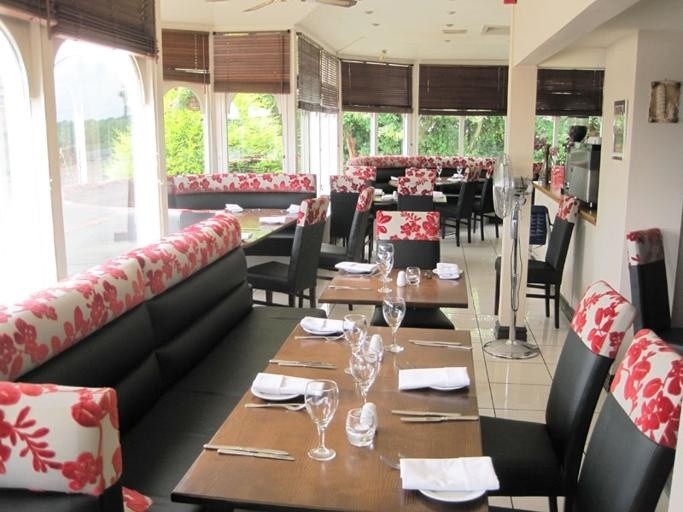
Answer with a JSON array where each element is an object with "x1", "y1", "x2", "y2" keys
[
  {"x1": 482, "y1": 152, "x2": 540, "y2": 359},
  {"x1": 242, "y1": 0, "x2": 356, "y2": 12}
]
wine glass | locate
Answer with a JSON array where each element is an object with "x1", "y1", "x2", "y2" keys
[
  {"x1": 349, "y1": 348, "x2": 384, "y2": 408},
  {"x1": 382, "y1": 297, "x2": 407, "y2": 353},
  {"x1": 376, "y1": 242, "x2": 395, "y2": 283},
  {"x1": 375, "y1": 251, "x2": 395, "y2": 293},
  {"x1": 456, "y1": 165, "x2": 463, "y2": 177},
  {"x1": 342, "y1": 314, "x2": 370, "y2": 375},
  {"x1": 304, "y1": 379, "x2": 341, "y2": 462},
  {"x1": 436, "y1": 165, "x2": 443, "y2": 180}
]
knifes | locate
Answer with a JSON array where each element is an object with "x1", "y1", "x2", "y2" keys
[
  {"x1": 269, "y1": 359, "x2": 338, "y2": 370},
  {"x1": 203, "y1": 443, "x2": 289, "y2": 456},
  {"x1": 401, "y1": 415, "x2": 480, "y2": 423},
  {"x1": 217, "y1": 448, "x2": 296, "y2": 461},
  {"x1": 408, "y1": 339, "x2": 474, "y2": 350},
  {"x1": 391, "y1": 409, "x2": 462, "y2": 417}
]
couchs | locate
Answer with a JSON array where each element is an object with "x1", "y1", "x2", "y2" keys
[
  {"x1": 164, "y1": 171, "x2": 317, "y2": 256},
  {"x1": 0, "y1": 214, "x2": 328, "y2": 512}
]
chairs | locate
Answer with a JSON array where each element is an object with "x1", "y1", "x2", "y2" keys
[
  {"x1": 434, "y1": 167, "x2": 480, "y2": 246},
  {"x1": 316, "y1": 186, "x2": 374, "y2": 279},
  {"x1": 474, "y1": 161, "x2": 494, "y2": 240},
  {"x1": 533, "y1": 162, "x2": 543, "y2": 205},
  {"x1": 328, "y1": 176, "x2": 368, "y2": 247},
  {"x1": 369, "y1": 210, "x2": 454, "y2": 329},
  {"x1": 573, "y1": 328, "x2": 683, "y2": 512},
  {"x1": 480, "y1": 280, "x2": 638, "y2": 512},
  {"x1": 397, "y1": 177, "x2": 433, "y2": 212},
  {"x1": 626, "y1": 228, "x2": 683, "y2": 347},
  {"x1": 495, "y1": 195, "x2": 580, "y2": 329},
  {"x1": 246, "y1": 196, "x2": 328, "y2": 309},
  {"x1": 342, "y1": 158, "x2": 496, "y2": 191}
]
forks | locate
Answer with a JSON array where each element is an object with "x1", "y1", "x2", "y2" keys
[
  {"x1": 294, "y1": 333, "x2": 345, "y2": 341},
  {"x1": 245, "y1": 401, "x2": 306, "y2": 411}
]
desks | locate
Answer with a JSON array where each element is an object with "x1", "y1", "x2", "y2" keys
[{"x1": 317, "y1": 267, "x2": 469, "y2": 328}]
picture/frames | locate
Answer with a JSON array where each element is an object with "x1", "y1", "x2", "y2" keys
[{"x1": 611, "y1": 99, "x2": 628, "y2": 157}]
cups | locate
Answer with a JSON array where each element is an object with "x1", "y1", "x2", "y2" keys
[
  {"x1": 345, "y1": 408, "x2": 379, "y2": 448},
  {"x1": 405, "y1": 266, "x2": 421, "y2": 286}
]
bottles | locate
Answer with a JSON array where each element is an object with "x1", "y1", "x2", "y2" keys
[
  {"x1": 368, "y1": 334, "x2": 383, "y2": 351},
  {"x1": 396, "y1": 271, "x2": 408, "y2": 287}
]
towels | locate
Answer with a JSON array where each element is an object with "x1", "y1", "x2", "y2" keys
[
  {"x1": 252, "y1": 373, "x2": 325, "y2": 397},
  {"x1": 399, "y1": 366, "x2": 470, "y2": 391},
  {"x1": 400, "y1": 456, "x2": 500, "y2": 491}
]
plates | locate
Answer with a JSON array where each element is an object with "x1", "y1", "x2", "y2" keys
[
  {"x1": 250, "y1": 389, "x2": 299, "y2": 401},
  {"x1": 429, "y1": 386, "x2": 468, "y2": 391},
  {"x1": 418, "y1": 490, "x2": 488, "y2": 504},
  {"x1": 302, "y1": 326, "x2": 336, "y2": 336}
]
